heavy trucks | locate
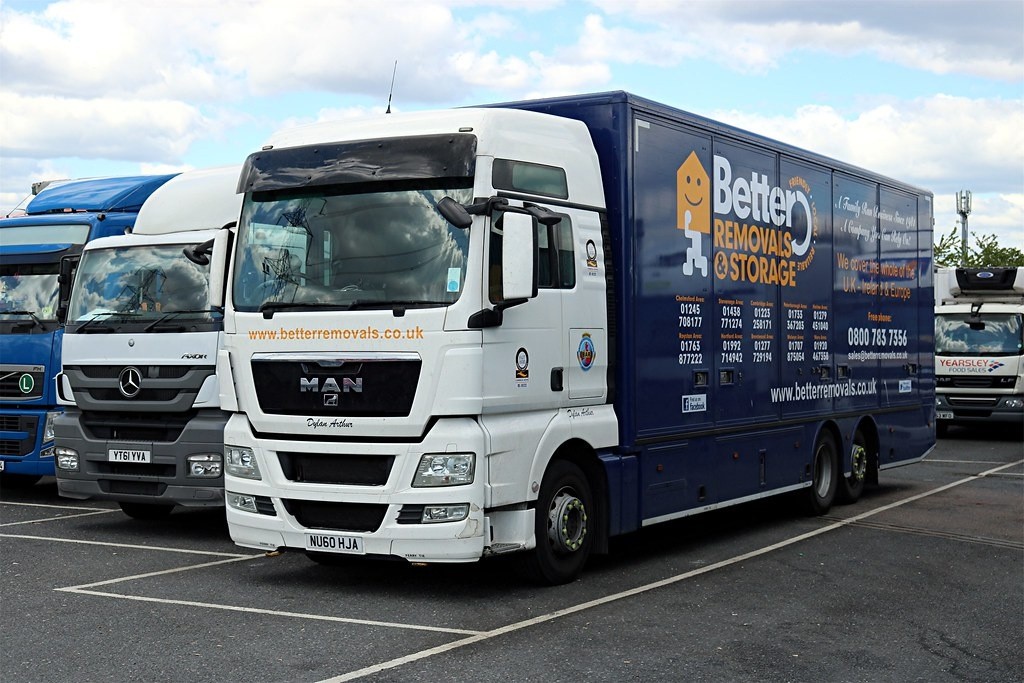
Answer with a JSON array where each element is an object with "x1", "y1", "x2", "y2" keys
[{"x1": 202, "y1": 88, "x2": 938, "y2": 586}]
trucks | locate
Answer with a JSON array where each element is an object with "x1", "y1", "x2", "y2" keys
[
  {"x1": 928, "y1": 264, "x2": 1024, "y2": 438},
  {"x1": 0, "y1": 172, "x2": 296, "y2": 494},
  {"x1": 45, "y1": 162, "x2": 330, "y2": 530}
]
